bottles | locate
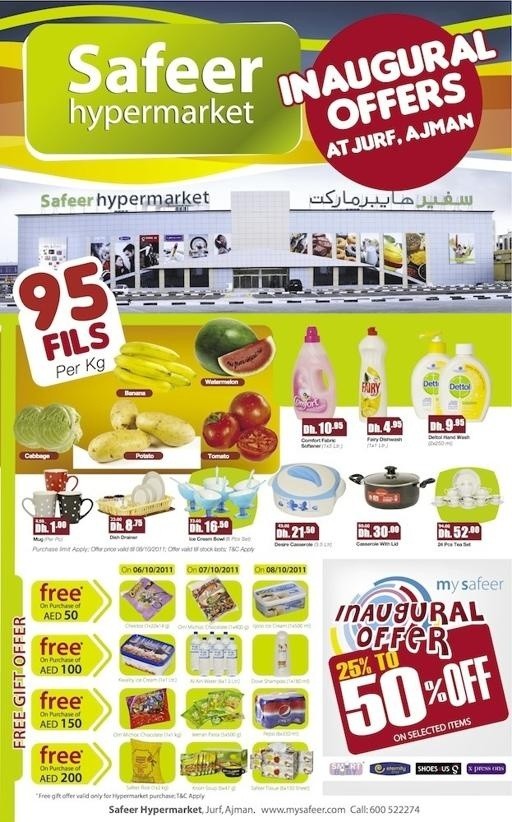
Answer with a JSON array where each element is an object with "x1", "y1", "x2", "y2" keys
[{"x1": 190, "y1": 630, "x2": 238, "y2": 679}]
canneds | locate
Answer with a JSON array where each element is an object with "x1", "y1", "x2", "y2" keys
[{"x1": 255, "y1": 692, "x2": 305, "y2": 729}]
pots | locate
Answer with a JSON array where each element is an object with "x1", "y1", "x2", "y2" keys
[
  {"x1": 350, "y1": 466, "x2": 435, "y2": 510},
  {"x1": 190, "y1": 246, "x2": 208, "y2": 258}
]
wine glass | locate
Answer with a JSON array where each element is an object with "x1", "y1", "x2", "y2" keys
[{"x1": 178, "y1": 477, "x2": 261, "y2": 519}]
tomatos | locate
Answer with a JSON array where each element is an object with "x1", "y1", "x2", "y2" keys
[{"x1": 203, "y1": 390, "x2": 278, "y2": 460}]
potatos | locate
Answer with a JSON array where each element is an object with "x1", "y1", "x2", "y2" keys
[{"x1": 88, "y1": 400, "x2": 195, "y2": 462}]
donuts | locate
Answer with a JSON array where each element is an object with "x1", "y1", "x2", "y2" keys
[{"x1": 336, "y1": 233, "x2": 356, "y2": 261}]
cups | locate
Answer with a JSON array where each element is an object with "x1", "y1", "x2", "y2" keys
[
  {"x1": 22, "y1": 470, "x2": 93, "y2": 525},
  {"x1": 365, "y1": 239, "x2": 378, "y2": 268},
  {"x1": 434, "y1": 488, "x2": 502, "y2": 507}
]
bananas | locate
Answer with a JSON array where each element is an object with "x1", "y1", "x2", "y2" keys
[{"x1": 113, "y1": 341, "x2": 196, "y2": 392}]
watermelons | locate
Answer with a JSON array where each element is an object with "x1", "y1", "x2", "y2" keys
[{"x1": 194, "y1": 319, "x2": 277, "y2": 377}]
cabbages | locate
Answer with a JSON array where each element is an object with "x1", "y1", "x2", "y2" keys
[{"x1": 11, "y1": 402, "x2": 83, "y2": 454}]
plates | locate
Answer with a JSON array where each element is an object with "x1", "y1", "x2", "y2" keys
[
  {"x1": 453, "y1": 469, "x2": 481, "y2": 496},
  {"x1": 131, "y1": 472, "x2": 166, "y2": 507},
  {"x1": 190, "y1": 236, "x2": 208, "y2": 250}
]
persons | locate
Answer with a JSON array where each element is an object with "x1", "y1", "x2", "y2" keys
[
  {"x1": 122, "y1": 244, "x2": 135, "y2": 272},
  {"x1": 114, "y1": 254, "x2": 129, "y2": 276},
  {"x1": 215, "y1": 234, "x2": 231, "y2": 255},
  {"x1": 99, "y1": 246, "x2": 110, "y2": 270}
]
